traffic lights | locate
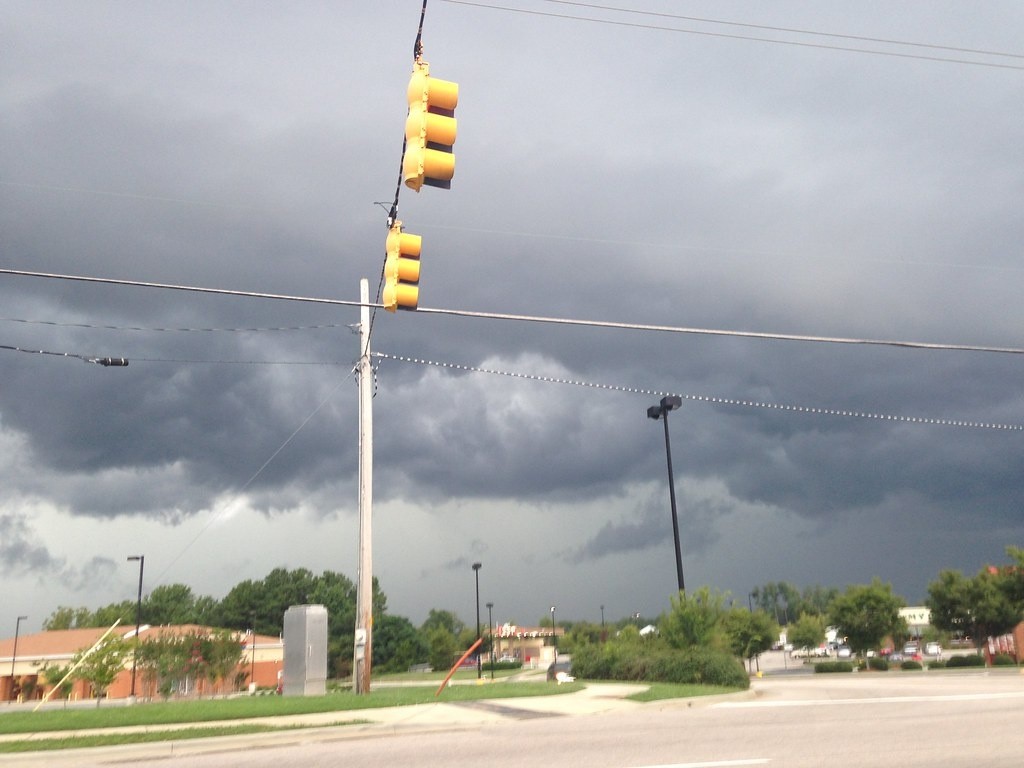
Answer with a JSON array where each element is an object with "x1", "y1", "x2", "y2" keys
[
  {"x1": 381, "y1": 219, "x2": 422, "y2": 312},
  {"x1": 401, "y1": 58, "x2": 458, "y2": 192}
]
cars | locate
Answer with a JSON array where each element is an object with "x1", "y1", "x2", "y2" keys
[
  {"x1": 925, "y1": 642, "x2": 942, "y2": 656},
  {"x1": 903, "y1": 641, "x2": 919, "y2": 656},
  {"x1": 965, "y1": 636, "x2": 974, "y2": 642},
  {"x1": 879, "y1": 642, "x2": 892, "y2": 656},
  {"x1": 837, "y1": 645, "x2": 852, "y2": 659},
  {"x1": 790, "y1": 646, "x2": 826, "y2": 658},
  {"x1": 862, "y1": 649, "x2": 879, "y2": 658}
]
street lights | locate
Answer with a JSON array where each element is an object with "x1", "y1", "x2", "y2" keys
[
  {"x1": 550, "y1": 607, "x2": 559, "y2": 665},
  {"x1": 473, "y1": 563, "x2": 481, "y2": 679},
  {"x1": 486, "y1": 602, "x2": 494, "y2": 679},
  {"x1": 9, "y1": 615, "x2": 29, "y2": 703},
  {"x1": 249, "y1": 610, "x2": 256, "y2": 683},
  {"x1": 748, "y1": 593, "x2": 756, "y2": 613},
  {"x1": 127, "y1": 555, "x2": 145, "y2": 698},
  {"x1": 599, "y1": 604, "x2": 605, "y2": 627},
  {"x1": 647, "y1": 396, "x2": 687, "y2": 618}
]
